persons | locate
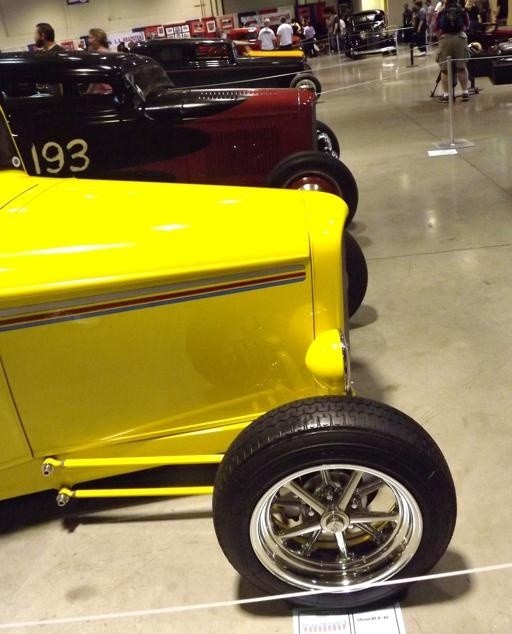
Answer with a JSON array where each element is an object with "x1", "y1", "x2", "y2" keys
[
  {"x1": 403, "y1": 1, "x2": 509, "y2": 101},
  {"x1": 303, "y1": 21, "x2": 316, "y2": 58},
  {"x1": 325, "y1": 6, "x2": 353, "y2": 55},
  {"x1": 85, "y1": 28, "x2": 115, "y2": 96},
  {"x1": 276, "y1": 15, "x2": 294, "y2": 50},
  {"x1": 257, "y1": 17, "x2": 277, "y2": 51},
  {"x1": 33, "y1": 22, "x2": 67, "y2": 96},
  {"x1": 301, "y1": 18, "x2": 316, "y2": 55},
  {"x1": 288, "y1": 19, "x2": 301, "y2": 35}
]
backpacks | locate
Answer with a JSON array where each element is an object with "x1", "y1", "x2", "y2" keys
[{"x1": 439, "y1": 8, "x2": 465, "y2": 35}]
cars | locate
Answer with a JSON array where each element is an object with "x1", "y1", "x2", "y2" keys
[{"x1": 338, "y1": 9, "x2": 398, "y2": 60}]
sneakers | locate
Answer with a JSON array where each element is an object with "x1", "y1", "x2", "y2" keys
[
  {"x1": 462, "y1": 93, "x2": 470, "y2": 101},
  {"x1": 439, "y1": 96, "x2": 456, "y2": 103}
]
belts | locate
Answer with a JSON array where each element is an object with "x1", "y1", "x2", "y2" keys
[{"x1": 438, "y1": 30, "x2": 466, "y2": 37}]
